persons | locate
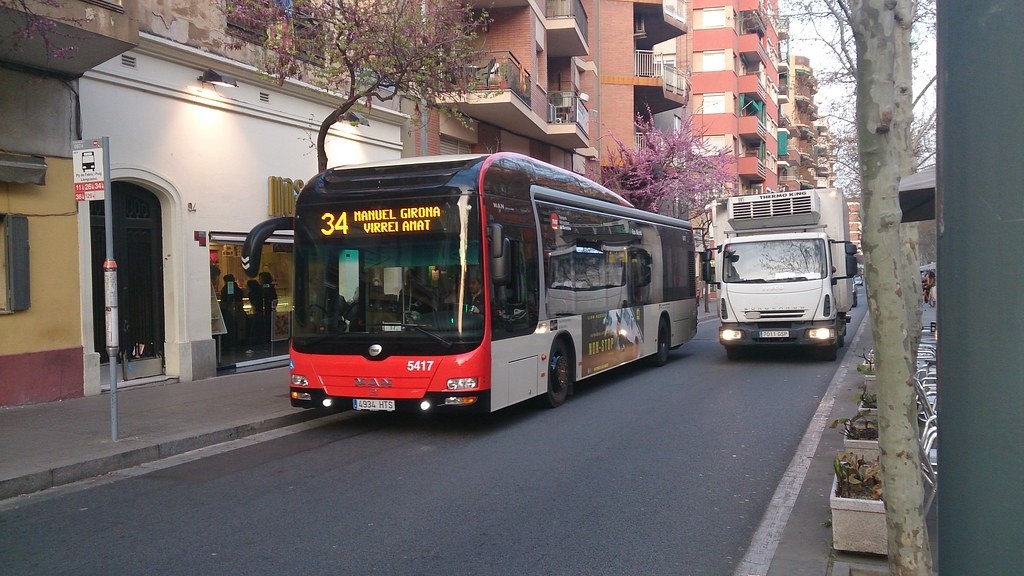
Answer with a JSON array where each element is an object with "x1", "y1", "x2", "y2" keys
[
  {"x1": 338, "y1": 287, "x2": 359, "y2": 332},
  {"x1": 221, "y1": 272, "x2": 278, "y2": 355},
  {"x1": 923, "y1": 271, "x2": 936, "y2": 302},
  {"x1": 398, "y1": 270, "x2": 433, "y2": 314},
  {"x1": 696, "y1": 276, "x2": 702, "y2": 306},
  {"x1": 464, "y1": 277, "x2": 484, "y2": 314}
]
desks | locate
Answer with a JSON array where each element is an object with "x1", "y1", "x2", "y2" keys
[{"x1": 462, "y1": 65, "x2": 482, "y2": 83}]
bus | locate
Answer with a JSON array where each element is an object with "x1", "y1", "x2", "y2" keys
[{"x1": 239, "y1": 150, "x2": 697, "y2": 419}]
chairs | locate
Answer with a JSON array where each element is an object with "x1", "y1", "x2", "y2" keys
[{"x1": 464, "y1": 57, "x2": 500, "y2": 87}]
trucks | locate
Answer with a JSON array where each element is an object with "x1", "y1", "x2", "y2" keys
[{"x1": 701, "y1": 185, "x2": 858, "y2": 362}]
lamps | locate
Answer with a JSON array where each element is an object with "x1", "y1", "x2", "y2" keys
[
  {"x1": 589, "y1": 108, "x2": 599, "y2": 117},
  {"x1": 197, "y1": 69, "x2": 239, "y2": 88},
  {"x1": 573, "y1": 93, "x2": 589, "y2": 102}
]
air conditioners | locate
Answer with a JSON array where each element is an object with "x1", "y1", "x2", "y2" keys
[
  {"x1": 548, "y1": 91, "x2": 572, "y2": 108},
  {"x1": 635, "y1": 14, "x2": 646, "y2": 37}
]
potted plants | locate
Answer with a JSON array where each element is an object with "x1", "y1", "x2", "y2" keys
[
  {"x1": 830, "y1": 409, "x2": 879, "y2": 463},
  {"x1": 856, "y1": 380, "x2": 877, "y2": 414},
  {"x1": 852, "y1": 344, "x2": 876, "y2": 392},
  {"x1": 830, "y1": 453, "x2": 888, "y2": 554}
]
